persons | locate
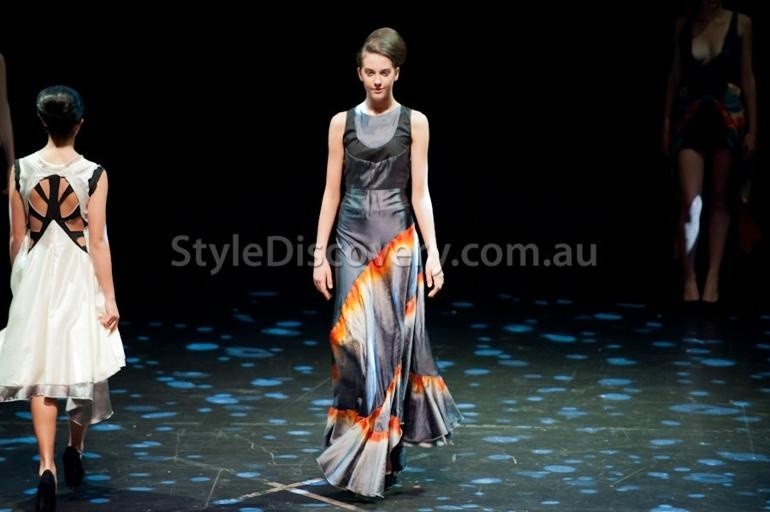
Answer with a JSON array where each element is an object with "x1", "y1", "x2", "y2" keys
[
  {"x1": 311, "y1": 27, "x2": 465, "y2": 498},
  {"x1": 658, "y1": 0, "x2": 757, "y2": 320},
  {"x1": 0, "y1": 56, "x2": 16, "y2": 197},
  {"x1": 1, "y1": 84, "x2": 129, "y2": 512}
]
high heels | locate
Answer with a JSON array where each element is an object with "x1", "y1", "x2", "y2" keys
[
  {"x1": 37, "y1": 470, "x2": 56, "y2": 512},
  {"x1": 63, "y1": 446, "x2": 84, "y2": 488}
]
